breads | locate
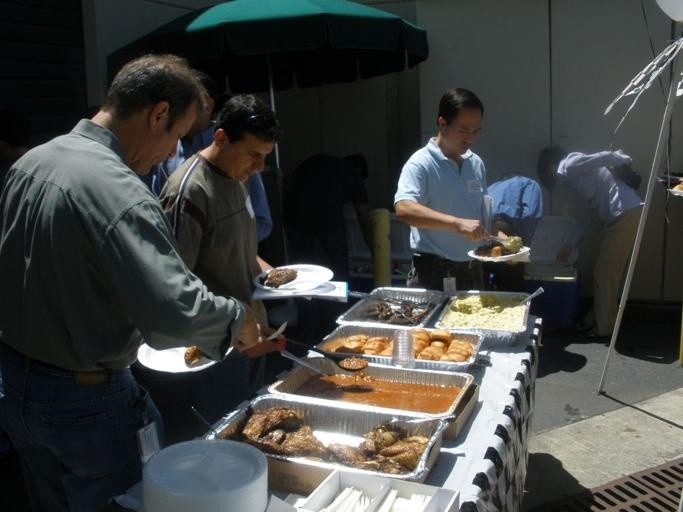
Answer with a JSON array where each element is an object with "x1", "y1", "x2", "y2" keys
[
  {"x1": 491, "y1": 245, "x2": 502, "y2": 258},
  {"x1": 334, "y1": 329, "x2": 474, "y2": 361}
]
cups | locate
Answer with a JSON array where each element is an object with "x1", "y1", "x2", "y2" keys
[{"x1": 390, "y1": 327, "x2": 414, "y2": 368}]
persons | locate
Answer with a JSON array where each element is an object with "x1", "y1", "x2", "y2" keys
[
  {"x1": 537, "y1": 145, "x2": 645, "y2": 344},
  {"x1": 138, "y1": 73, "x2": 273, "y2": 242},
  {"x1": 393, "y1": 87, "x2": 494, "y2": 290},
  {"x1": 1, "y1": 56, "x2": 262, "y2": 510},
  {"x1": 2, "y1": 112, "x2": 36, "y2": 177},
  {"x1": 484, "y1": 171, "x2": 541, "y2": 291},
  {"x1": 158, "y1": 93, "x2": 286, "y2": 443}
]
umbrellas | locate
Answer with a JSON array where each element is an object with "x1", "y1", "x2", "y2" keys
[{"x1": 104, "y1": 0, "x2": 427, "y2": 259}]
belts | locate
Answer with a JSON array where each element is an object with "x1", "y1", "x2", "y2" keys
[{"x1": 438, "y1": 259, "x2": 482, "y2": 269}]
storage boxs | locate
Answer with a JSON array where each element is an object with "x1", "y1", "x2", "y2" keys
[
  {"x1": 490, "y1": 215, "x2": 581, "y2": 330},
  {"x1": 344, "y1": 210, "x2": 413, "y2": 308}
]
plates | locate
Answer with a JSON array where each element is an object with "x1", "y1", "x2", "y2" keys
[
  {"x1": 135, "y1": 340, "x2": 235, "y2": 377},
  {"x1": 249, "y1": 261, "x2": 333, "y2": 291},
  {"x1": 141, "y1": 440, "x2": 269, "y2": 512},
  {"x1": 467, "y1": 244, "x2": 532, "y2": 265}
]
